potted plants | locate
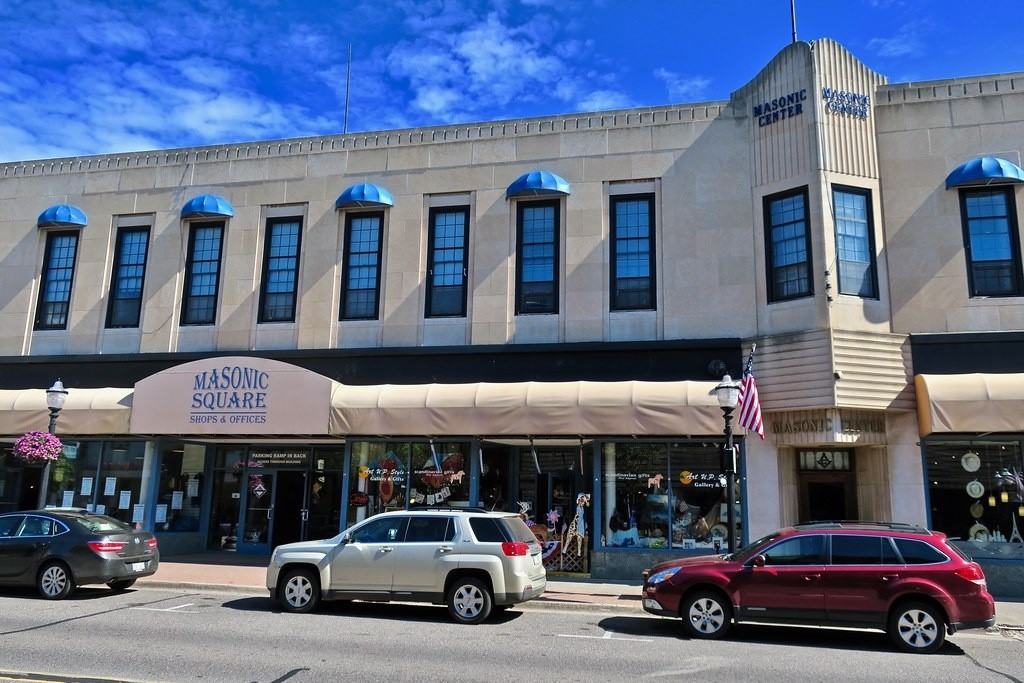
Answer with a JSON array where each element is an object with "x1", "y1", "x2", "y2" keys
[{"x1": 52, "y1": 455, "x2": 74, "y2": 483}]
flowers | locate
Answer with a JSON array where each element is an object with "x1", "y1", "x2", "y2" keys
[{"x1": 12, "y1": 431, "x2": 63, "y2": 461}]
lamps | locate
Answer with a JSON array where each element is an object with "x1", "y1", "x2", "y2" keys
[{"x1": 986, "y1": 445, "x2": 1024, "y2": 517}]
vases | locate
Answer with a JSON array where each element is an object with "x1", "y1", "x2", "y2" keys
[{"x1": 26, "y1": 459, "x2": 43, "y2": 468}]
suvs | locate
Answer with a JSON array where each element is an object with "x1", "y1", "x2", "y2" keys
[
  {"x1": 266, "y1": 506, "x2": 547, "y2": 625},
  {"x1": 642, "y1": 520, "x2": 997, "y2": 655}
]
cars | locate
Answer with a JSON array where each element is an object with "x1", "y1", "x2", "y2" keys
[{"x1": 0, "y1": 507, "x2": 160, "y2": 600}]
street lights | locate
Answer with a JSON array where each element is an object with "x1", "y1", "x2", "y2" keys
[
  {"x1": 36, "y1": 376, "x2": 70, "y2": 511},
  {"x1": 714, "y1": 369, "x2": 743, "y2": 555}
]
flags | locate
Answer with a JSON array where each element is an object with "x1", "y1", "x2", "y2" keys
[{"x1": 737, "y1": 342, "x2": 766, "y2": 440}]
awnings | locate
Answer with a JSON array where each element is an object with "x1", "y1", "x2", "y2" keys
[
  {"x1": 914, "y1": 373, "x2": 1024, "y2": 438},
  {"x1": 505, "y1": 170, "x2": 571, "y2": 200},
  {"x1": 181, "y1": 194, "x2": 234, "y2": 220},
  {"x1": 0, "y1": 387, "x2": 134, "y2": 435},
  {"x1": 335, "y1": 183, "x2": 394, "y2": 210},
  {"x1": 38, "y1": 204, "x2": 88, "y2": 228},
  {"x1": 946, "y1": 157, "x2": 1024, "y2": 191},
  {"x1": 329, "y1": 380, "x2": 727, "y2": 438}
]
